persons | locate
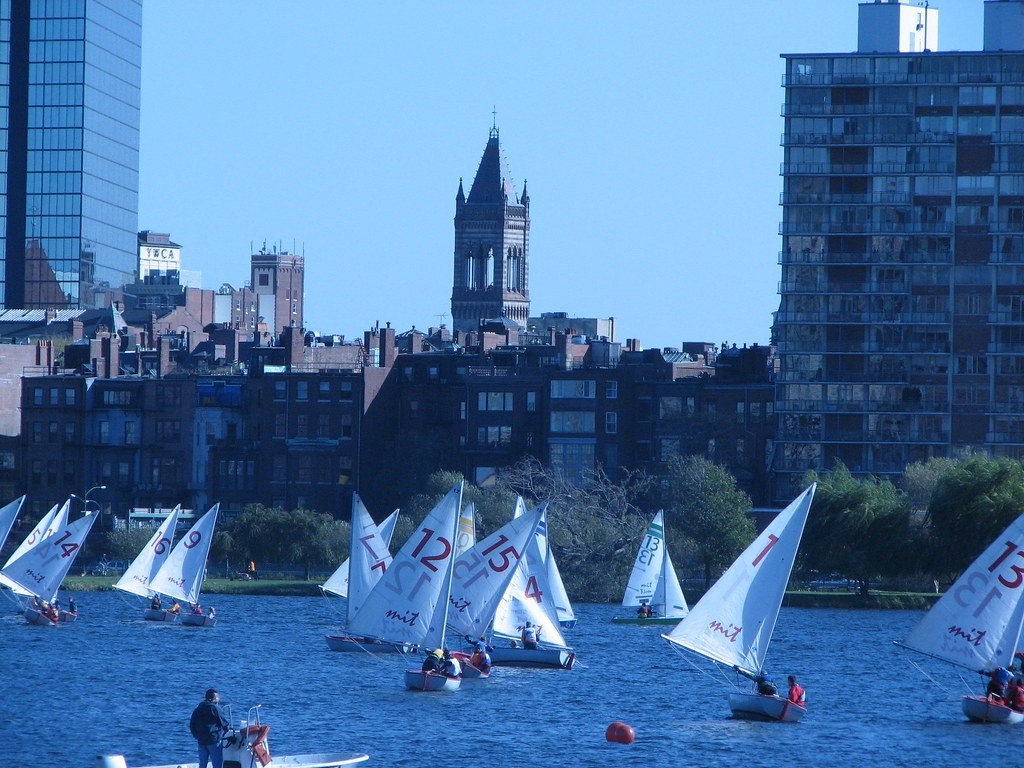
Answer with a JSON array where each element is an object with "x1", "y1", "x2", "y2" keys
[
  {"x1": 739, "y1": 669, "x2": 780, "y2": 695},
  {"x1": 250, "y1": 560, "x2": 256, "y2": 572},
  {"x1": 194, "y1": 604, "x2": 202, "y2": 615},
  {"x1": 209, "y1": 606, "x2": 216, "y2": 619},
  {"x1": 510, "y1": 641, "x2": 517, "y2": 648},
  {"x1": 979, "y1": 652, "x2": 1024, "y2": 712},
  {"x1": 637, "y1": 603, "x2": 656, "y2": 618},
  {"x1": 170, "y1": 599, "x2": 180, "y2": 615},
  {"x1": 422, "y1": 649, "x2": 463, "y2": 678},
  {"x1": 465, "y1": 635, "x2": 496, "y2": 674},
  {"x1": 787, "y1": 676, "x2": 805, "y2": 707},
  {"x1": 190, "y1": 689, "x2": 229, "y2": 768},
  {"x1": 151, "y1": 594, "x2": 161, "y2": 607},
  {"x1": 32, "y1": 596, "x2": 60, "y2": 621},
  {"x1": 69, "y1": 596, "x2": 78, "y2": 614},
  {"x1": 521, "y1": 621, "x2": 538, "y2": 650}
]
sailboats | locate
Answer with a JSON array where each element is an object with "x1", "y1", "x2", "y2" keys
[
  {"x1": 894, "y1": 513, "x2": 1024, "y2": 724},
  {"x1": 487, "y1": 494, "x2": 577, "y2": 671},
  {"x1": 344, "y1": 475, "x2": 461, "y2": 693},
  {"x1": 612, "y1": 509, "x2": 689, "y2": 624},
  {"x1": 419, "y1": 498, "x2": 547, "y2": 680},
  {"x1": 659, "y1": 481, "x2": 808, "y2": 722},
  {"x1": 111, "y1": 504, "x2": 179, "y2": 623},
  {"x1": 324, "y1": 508, "x2": 415, "y2": 653},
  {"x1": 0, "y1": 493, "x2": 100, "y2": 627},
  {"x1": 146, "y1": 502, "x2": 216, "y2": 627},
  {"x1": 536, "y1": 508, "x2": 578, "y2": 628}
]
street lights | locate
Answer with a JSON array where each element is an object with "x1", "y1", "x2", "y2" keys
[{"x1": 71, "y1": 486, "x2": 106, "y2": 551}]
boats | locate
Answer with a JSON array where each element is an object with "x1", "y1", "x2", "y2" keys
[{"x1": 97, "y1": 703, "x2": 369, "y2": 768}]
